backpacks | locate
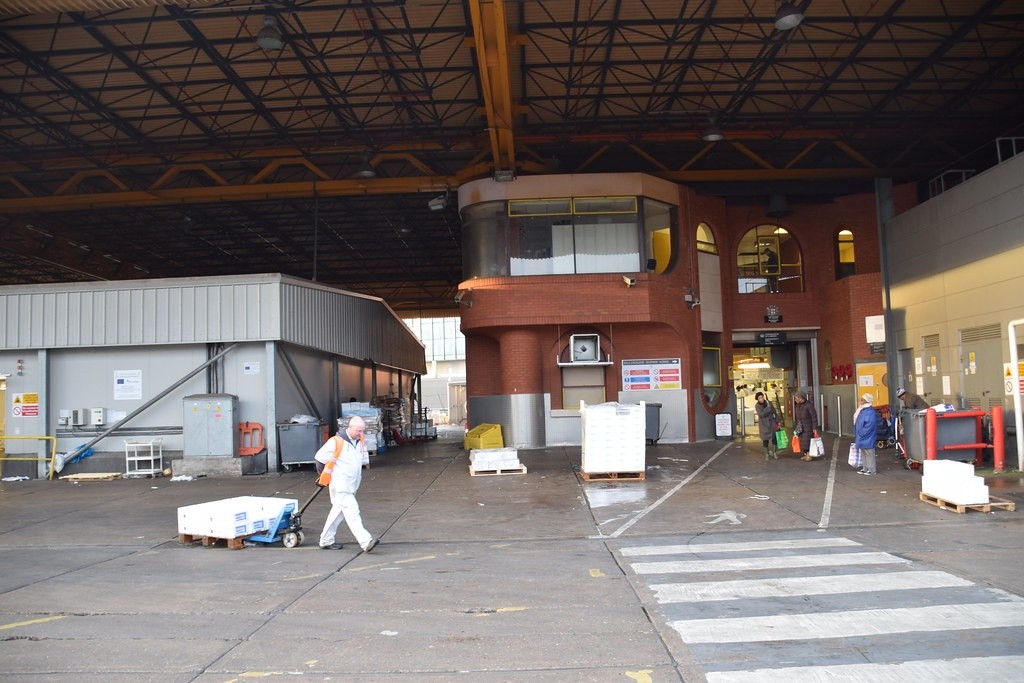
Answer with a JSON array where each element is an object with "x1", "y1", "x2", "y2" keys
[{"x1": 315, "y1": 436, "x2": 339, "y2": 477}]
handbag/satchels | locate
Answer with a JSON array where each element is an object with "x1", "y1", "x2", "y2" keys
[
  {"x1": 773, "y1": 418, "x2": 779, "y2": 430},
  {"x1": 809, "y1": 437, "x2": 825, "y2": 457},
  {"x1": 848, "y1": 443, "x2": 862, "y2": 468},
  {"x1": 775, "y1": 428, "x2": 789, "y2": 449},
  {"x1": 792, "y1": 433, "x2": 800, "y2": 453}
]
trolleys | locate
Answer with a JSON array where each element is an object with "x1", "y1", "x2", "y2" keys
[
  {"x1": 877, "y1": 412, "x2": 897, "y2": 448},
  {"x1": 243, "y1": 476, "x2": 325, "y2": 550},
  {"x1": 410, "y1": 408, "x2": 438, "y2": 441}
]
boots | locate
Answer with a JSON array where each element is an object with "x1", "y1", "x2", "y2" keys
[
  {"x1": 772, "y1": 444, "x2": 779, "y2": 460},
  {"x1": 763, "y1": 447, "x2": 770, "y2": 460}
]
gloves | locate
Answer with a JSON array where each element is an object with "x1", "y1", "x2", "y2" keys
[{"x1": 813, "y1": 431, "x2": 819, "y2": 438}]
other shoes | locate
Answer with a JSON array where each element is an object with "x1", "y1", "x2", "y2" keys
[
  {"x1": 800, "y1": 453, "x2": 812, "y2": 461},
  {"x1": 856, "y1": 470, "x2": 866, "y2": 475},
  {"x1": 365, "y1": 539, "x2": 380, "y2": 552},
  {"x1": 319, "y1": 543, "x2": 343, "y2": 549},
  {"x1": 864, "y1": 471, "x2": 876, "y2": 476}
]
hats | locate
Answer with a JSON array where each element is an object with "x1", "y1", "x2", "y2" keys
[
  {"x1": 862, "y1": 394, "x2": 873, "y2": 402},
  {"x1": 897, "y1": 388, "x2": 906, "y2": 397}
]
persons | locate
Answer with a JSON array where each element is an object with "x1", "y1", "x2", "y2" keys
[
  {"x1": 795, "y1": 390, "x2": 818, "y2": 462},
  {"x1": 853, "y1": 393, "x2": 878, "y2": 476},
  {"x1": 755, "y1": 392, "x2": 781, "y2": 461},
  {"x1": 764, "y1": 248, "x2": 779, "y2": 293},
  {"x1": 314, "y1": 416, "x2": 380, "y2": 553},
  {"x1": 896, "y1": 387, "x2": 930, "y2": 409}
]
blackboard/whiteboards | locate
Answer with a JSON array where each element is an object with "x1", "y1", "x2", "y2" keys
[{"x1": 716, "y1": 413, "x2": 732, "y2": 436}]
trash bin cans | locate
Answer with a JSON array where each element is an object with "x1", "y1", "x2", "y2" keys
[
  {"x1": 636, "y1": 403, "x2": 660, "y2": 443},
  {"x1": 901, "y1": 408, "x2": 977, "y2": 462}
]
